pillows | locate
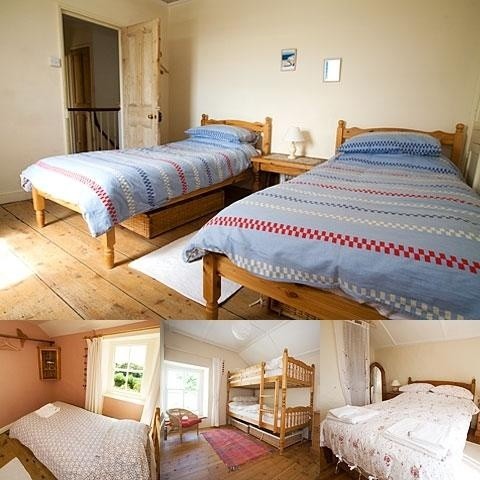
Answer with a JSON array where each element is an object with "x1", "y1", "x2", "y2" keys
[
  {"x1": 184, "y1": 124, "x2": 259, "y2": 145},
  {"x1": 398, "y1": 383, "x2": 435, "y2": 394},
  {"x1": 84, "y1": 419, "x2": 152, "y2": 480},
  {"x1": 337, "y1": 131, "x2": 443, "y2": 157},
  {"x1": 430, "y1": 385, "x2": 474, "y2": 400}
]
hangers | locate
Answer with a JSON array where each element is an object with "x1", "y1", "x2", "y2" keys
[{"x1": 0, "y1": 335, "x2": 21, "y2": 352}]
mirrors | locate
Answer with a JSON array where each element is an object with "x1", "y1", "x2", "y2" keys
[{"x1": 370, "y1": 362, "x2": 386, "y2": 404}]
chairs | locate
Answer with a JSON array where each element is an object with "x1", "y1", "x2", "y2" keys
[{"x1": 166, "y1": 408, "x2": 201, "y2": 443}]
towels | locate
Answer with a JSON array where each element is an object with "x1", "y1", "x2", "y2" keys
[
  {"x1": 34, "y1": 404, "x2": 61, "y2": 419},
  {"x1": 327, "y1": 405, "x2": 456, "y2": 460}
]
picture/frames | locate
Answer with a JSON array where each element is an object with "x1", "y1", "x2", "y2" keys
[
  {"x1": 322, "y1": 58, "x2": 341, "y2": 82},
  {"x1": 280, "y1": 48, "x2": 297, "y2": 71}
]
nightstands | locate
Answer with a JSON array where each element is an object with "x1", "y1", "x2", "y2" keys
[
  {"x1": 387, "y1": 390, "x2": 404, "y2": 400},
  {"x1": 250, "y1": 153, "x2": 328, "y2": 192}
]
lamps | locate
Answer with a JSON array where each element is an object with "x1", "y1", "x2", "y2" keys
[
  {"x1": 391, "y1": 379, "x2": 401, "y2": 391},
  {"x1": 283, "y1": 127, "x2": 304, "y2": 160}
]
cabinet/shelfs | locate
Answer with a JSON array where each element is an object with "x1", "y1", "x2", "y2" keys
[
  {"x1": 38, "y1": 345, "x2": 61, "y2": 381},
  {"x1": 312, "y1": 410, "x2": 319, "y2": 457}
]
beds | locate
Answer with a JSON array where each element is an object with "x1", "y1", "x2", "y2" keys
[
  {"x1": 226, "y1": 348, "x2": 315, "y2": 455},
  {"x1": 32, "y1": 114, "x2": 272, "y2": 269},
  {"x1": 325, "y1": 377, "x2": 476, "y2": 479},
  {"x1": 9, "y1": 400, "x2": 160, "y2": 480},
  {"x1": 204, "y1": 119, "x2": 479, "y2": 320}
]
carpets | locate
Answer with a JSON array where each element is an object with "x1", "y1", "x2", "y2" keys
[
  {"x1": 459, "y1": 441, "x2": 479, "y2": 479},
  {"x1": 129, "y1": 229, "x2": 244, "y2": 309},
  {"x1": 202, "y1": 421, "x2": 277, "y2": 469},
  {"x1": 0, "y1": 457, "x2": 33, "y2": 480}
]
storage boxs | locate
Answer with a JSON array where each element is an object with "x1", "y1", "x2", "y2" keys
[
  {"x1": 231, "y1": 418, "x2": 249, "y2": 434},
  {"x1": 250, "y1": 425, "x2": 305, "y2": 450},
  {"x1": 118, "y1": 190, "x2": 225, "y2": 240}
]
